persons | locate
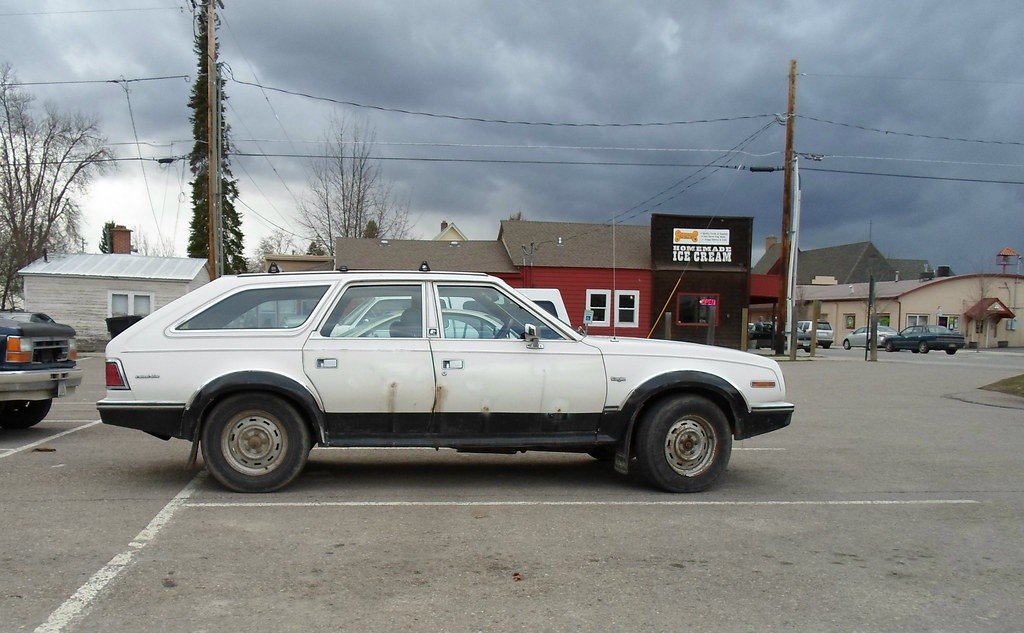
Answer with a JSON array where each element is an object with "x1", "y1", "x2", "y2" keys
[{"x1": 755, "y1": 316, "x2": 764, "y2": 350}]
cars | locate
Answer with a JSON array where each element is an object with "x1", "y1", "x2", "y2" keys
[
  {"x1": 1, "y1": 309, "x2": 82, "y2": 426},
  {"x1": 881, "y1": 324, "x2": 965, "y2": 355},
  {"x1": 843, "y1": 325, "x2": 897, "y2": 350},
  {"x1": 338, "y1": 307, "x2": 566, "y2": 339}
]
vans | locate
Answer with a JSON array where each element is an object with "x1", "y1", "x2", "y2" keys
[
  {"x1": 284, "y1": 287, "x2": 573, "y2": 341},
  {"x1": 747, "y1": 323, "x2": 756, "y2": 338},
  {"x1": 756, "y1": 320, "x2": 811, "y2": 352},
  {"x1": 798, "y1": 318, "x2": 834, "y2": 349}
]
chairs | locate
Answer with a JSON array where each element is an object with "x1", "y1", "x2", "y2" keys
[{"x1": 389, "y1": 308, "x2": 422, "y2": 337}]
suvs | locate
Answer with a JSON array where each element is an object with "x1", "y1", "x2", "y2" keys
[{"x1": 96, "y1": 261, "x2": 795, "y2": 495}]
